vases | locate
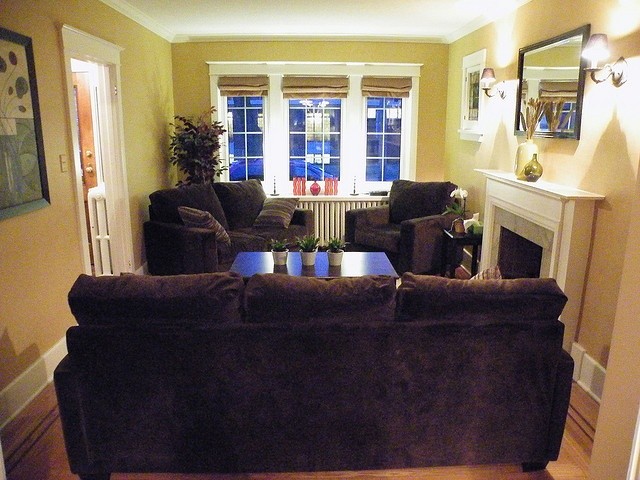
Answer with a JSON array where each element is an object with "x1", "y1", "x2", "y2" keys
[
  {"x1": 310, "y1": 179, "x2": 321, "y2": 195},
  {"x1": 516, "y1": 141, "x2": 538, "y2": 179},
  {"x1": 523, "y1": 154, "x2": 543, "y2": 183}
]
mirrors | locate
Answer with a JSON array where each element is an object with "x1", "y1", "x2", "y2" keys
[{"x1": 513, "y1": 24, "x2": 591, "y2": 140}]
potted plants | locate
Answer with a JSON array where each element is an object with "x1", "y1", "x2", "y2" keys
[
  {"x1": 442, "y1": 186, "x2": 469, "y2": 234},
  {"x1": 325, "y1": 236, "x2": 345, "y2": 266},
  {"x1": 268, "y1": 238, "x2": 290, "y2": 265},
  {"x1": 294, "y1": 232, "x2": 322, "y2": 266}
]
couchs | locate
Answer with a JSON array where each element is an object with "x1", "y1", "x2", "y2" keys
[
  {"x1": 344, "y1": 180, "x2": 464, "y2": 273},
  {"x1": 142, "y1": 180, "x2": 314, "y2": 276},
  {"x1": 52, "y1": 272, "x2": 576, "y2": 480}
]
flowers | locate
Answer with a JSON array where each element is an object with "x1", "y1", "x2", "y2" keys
[
  {"x1": 520, "y1": 97, "x2": 547, "y2": 142},
  {"x1": 546, "y1": 99, "x2": 576, "y2": 131}
]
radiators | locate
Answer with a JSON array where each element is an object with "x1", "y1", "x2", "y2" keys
[{"x1": 273, "y1": 193, "x2": 389, "y2": 247}]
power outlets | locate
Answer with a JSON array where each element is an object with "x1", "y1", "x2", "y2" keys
[{"x1": 59, "y1": 154, "x2": 70, "y2": 172}]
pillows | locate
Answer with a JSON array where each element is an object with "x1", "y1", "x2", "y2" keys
[
  {"x1": 253, "y1": 196, "x2": 300, "y2": 229},
  {"x1": 178, "y1": 204, "x2": 232, "y2": 245}
]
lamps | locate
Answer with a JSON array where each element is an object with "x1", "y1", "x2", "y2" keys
[
  {"x1": 482, "y1": 66, "x2": 508, "y2": 99},
  {"x1": 579, "y1": 32, "x2": 628, "y2": 87}
]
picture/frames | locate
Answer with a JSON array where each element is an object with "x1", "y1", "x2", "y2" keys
[
  {"x1": 457, "y1": 48, "x2": 486, "y2": 140},
  {"x1": 0, "y1": 27, "x2": 52, "y2": 222}
]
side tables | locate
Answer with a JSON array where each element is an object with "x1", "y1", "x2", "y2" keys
[{"x1": 440, "y1": 230, "x2": 483, "y2": 278}]
